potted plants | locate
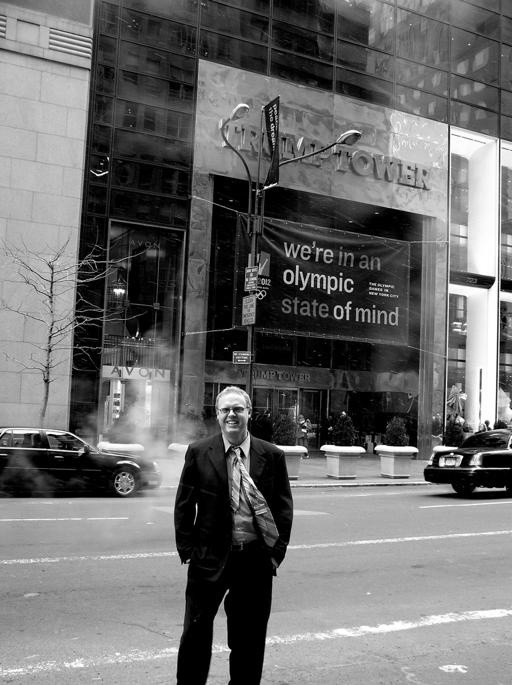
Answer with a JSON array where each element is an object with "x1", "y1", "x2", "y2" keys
[
  {"x1": 320, "y1": 411, "x2": 367, "y2": 480},
  {"x1": 97, "y1": 409, "x2": 144, "y2": 459},
  {"x1": 374, "y1": 415, "x2": 419, "y2": 479},
  {"x1": 269, "y1": 406, "x2": 309, "y2": 480},
  {"x1": 168, "y1": 403, "x2": 209, "y2": 480}
]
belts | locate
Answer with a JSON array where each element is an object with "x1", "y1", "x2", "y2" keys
[{"x1": 231, "y1": 543, "x2": 243, "y2": 552}]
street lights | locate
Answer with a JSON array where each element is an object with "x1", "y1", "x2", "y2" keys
[{"x1": 221, "y1": 104, "x2": 362, "y2": 435}]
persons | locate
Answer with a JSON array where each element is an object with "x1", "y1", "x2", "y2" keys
[
  {"x1": 296, "y1": 411, "x2": 354, "y2": 460},
  {"x1": 174, "y1": 385, "x2": 294, "y2": 685},
  {"x1": 430, "y1": 412, "x2": 507, "y2": 447}
]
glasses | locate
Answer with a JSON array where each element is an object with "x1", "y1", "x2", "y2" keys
[{"x1": 217, "y1": 407, "x2": 249, "y2": 414}]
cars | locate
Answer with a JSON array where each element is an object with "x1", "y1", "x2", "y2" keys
[
  {"x1": 423, "y1": 429, "x2": 512, "y2": 493},
  {"x1": 0, "y1": 427, "x2": 163, "y2": 498}
]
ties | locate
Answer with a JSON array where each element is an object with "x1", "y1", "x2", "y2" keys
[{"x1": 230, "y1": 448, "x2": 279, "y2": 547}]
